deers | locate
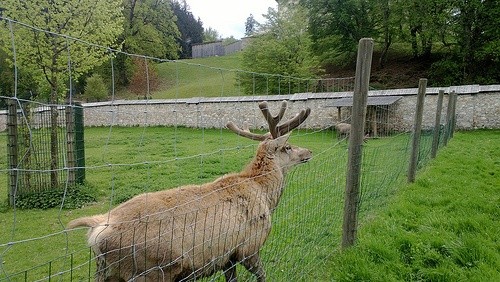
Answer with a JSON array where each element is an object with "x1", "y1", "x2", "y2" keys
[
  {"x1": 63, "y1": 99, "x2": 314, "y2": 282},
  {"x1": 333, "y1": 120, "x2": 370, "y2": 146}
]
